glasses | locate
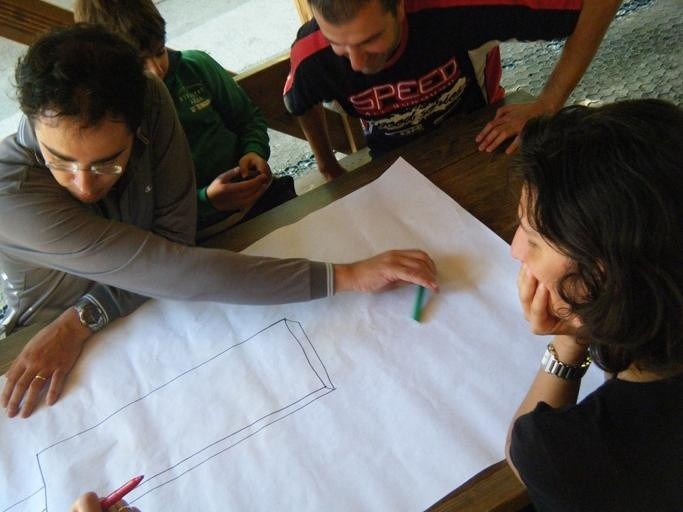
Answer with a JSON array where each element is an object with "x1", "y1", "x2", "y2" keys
[{"x1": 31, "y1": 133, "x2": 123, "y2": 177}]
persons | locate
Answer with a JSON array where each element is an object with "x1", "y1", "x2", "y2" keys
[
  {"x1": 283, "y1": 1, "x2": 623, "y2": 179},
  {"x1": 69, "y1": 0, "x2": 298, "y2": 242},
  {"x1": 0, "y1": 21, "x2": 443, "y2": 420},
  {"x1": 502, "y1": 99, "x2": 682, "y2": 512},
  {"x1": 71, "y1": 492, "x2": 141, "y2": 512}
]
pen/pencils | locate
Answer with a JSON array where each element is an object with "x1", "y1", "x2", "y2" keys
[
  {"x1": 101, "y1": 475, "x2": 143, "y2": 511},
  {"x1": 412, "y1": 284, "x2": 423, "y2": 321}
]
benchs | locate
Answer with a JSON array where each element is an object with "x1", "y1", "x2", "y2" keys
[{"x1": 227, "y1": 40, "x2": 368, "y2": 153}]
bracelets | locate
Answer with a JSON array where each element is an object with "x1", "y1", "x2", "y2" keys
[{"x1": 543, "y1": 344, "x2": 592, "y2": 379}]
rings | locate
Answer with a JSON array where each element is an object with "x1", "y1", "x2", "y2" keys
[{"x1": 34, "y1": 375, "x2": 46, "y2": 380}]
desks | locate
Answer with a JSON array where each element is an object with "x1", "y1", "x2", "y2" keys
[{"x1": 0, "y1": 87, "x2": 617, "y2": 512}]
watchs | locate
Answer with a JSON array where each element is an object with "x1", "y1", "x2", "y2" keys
[{"x1": 74, "y1": 298, "x2": 104, "y2": 334}]
